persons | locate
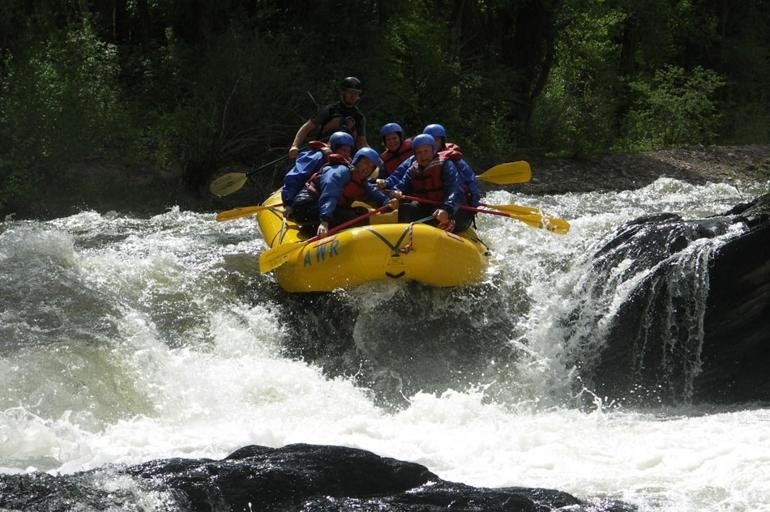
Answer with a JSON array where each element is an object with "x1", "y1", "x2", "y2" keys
[
  {"x1": 377, "y1": 122, "x2": 416, "y2": 179},
  {"x1": 389, "y1": 132, "x2": 466, "y2": 225},
  {"x1": 281, "y1": 131, "x2": 355, "y2": 222},
  {"x1": 289, "y1": 77, "x2": 370, "y2": 161},
  {"x1": 293, "y1": 146, "x2": 400, "y2": 240},
  {"x1": 376, "y1": 123, "x2": 480, "y2": 233}
]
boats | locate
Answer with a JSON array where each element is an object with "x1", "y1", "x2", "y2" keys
[{"x1": 259, "y1": 185, "x2": 487, "y2": 294}]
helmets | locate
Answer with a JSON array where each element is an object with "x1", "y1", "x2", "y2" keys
[
  {"x1": 351, "y1": 147, "x2": 380, "y2": 170},
  {"x1": 329, "y1": 132, "x2": 354, "y2": 149},
  {"x1": 412, "y1": 134, "x2": 435, "y2": 153},
  {"x1": 340, "y1": 76, "x2": 362, "y2": 94},
  {"x1": 423, "y1": 124, "x2": 447, "y2": 139},
  {"x1": 380, "y1": 123, "x2": 404, "y2": 146}
]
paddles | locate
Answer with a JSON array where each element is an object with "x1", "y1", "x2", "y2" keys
[
  {"x1": 214, "y1": 201, "x2": 283, "y2": 223},
  {"x1": 259, "y1": 203, "x2": 388, "y2": 273},
  {"x1": 475, "y1": 161, "x2": 531, "y2": 184},
  {"x1": 210, "y1": 134, "x2": 331, "y2": 198},
  {"x1": 397, "y1": 194, "x2": 570, "y2": 235},
  {"x1": 476, "y1": 202, "x2": 540, "y2": 217}
]
